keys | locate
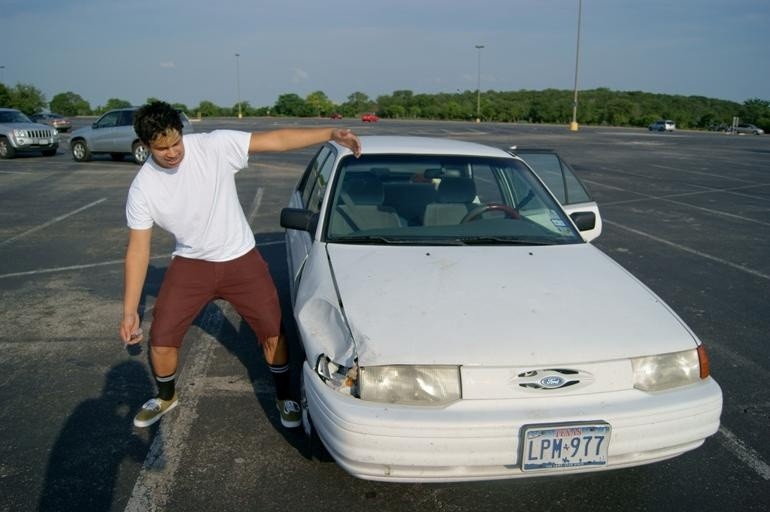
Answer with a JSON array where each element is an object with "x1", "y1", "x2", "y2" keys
[{"x1": 123, "y1": 327, "x2": 144, "y2": 351}]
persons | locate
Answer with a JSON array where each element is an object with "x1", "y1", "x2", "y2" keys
[{"x1": 119, "y1": 98, "x2": 363, "y2": 431}]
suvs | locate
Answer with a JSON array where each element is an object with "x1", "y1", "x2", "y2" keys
[{"x1": 1, "y1": 108, "x2": 59, "y2": 155}]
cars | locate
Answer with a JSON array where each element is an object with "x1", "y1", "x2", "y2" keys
[
  {"x1": 649, "y1": 120, "x2": 675, "y2": 131},
  {"x1": 707, "y1": 122, "x2": 764, "y2": 135},
  {"x1": 32, "y1": 112, "x2": 71, "y2": 132},
  {"x1": 69, "y1": 108, "x2": 194, "y2": 164},
  {"x1": 280, "y1": 136, "x2": 722, "y2": 483},
  {"x1": 331, "y1": 112, "x2": 342, "y2": 120},
  {"x1": 362, "y1": 114, "x2": 379, "y2": 123}
]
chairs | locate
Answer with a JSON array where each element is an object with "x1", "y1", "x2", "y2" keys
[{"x1": 331, "y1": 167, "x2": 488, "y2": 234}]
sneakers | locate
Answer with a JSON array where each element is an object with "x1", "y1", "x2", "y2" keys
[
  {"x1": 133, "y1": 391, "x2": 178, "y2": 428},
  {"x1": 275, "y1": 397, "x2": 302, "y2": 428}
]
